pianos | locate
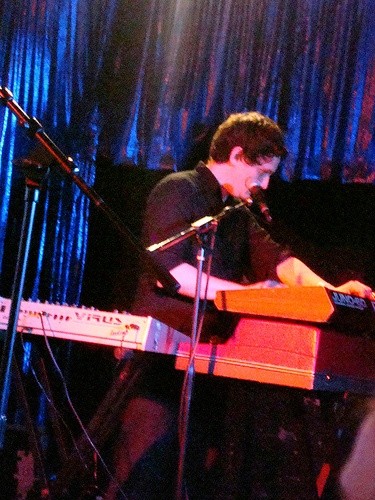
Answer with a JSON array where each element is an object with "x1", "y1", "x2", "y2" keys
[
  {"x1": 0, "y1": 295, "x2": 194, "y2": 357},
  {"x1": 172, "y1": 282, "x2": 374, "y2": 394}
]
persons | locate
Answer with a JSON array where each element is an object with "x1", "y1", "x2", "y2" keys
[{"x1": 93, "y1": 112, "x2": 375, "y2": 500}]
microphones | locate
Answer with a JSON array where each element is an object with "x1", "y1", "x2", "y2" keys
[{"x1": 249, "y1": 186, "x2": 272, "y2": 222}]
